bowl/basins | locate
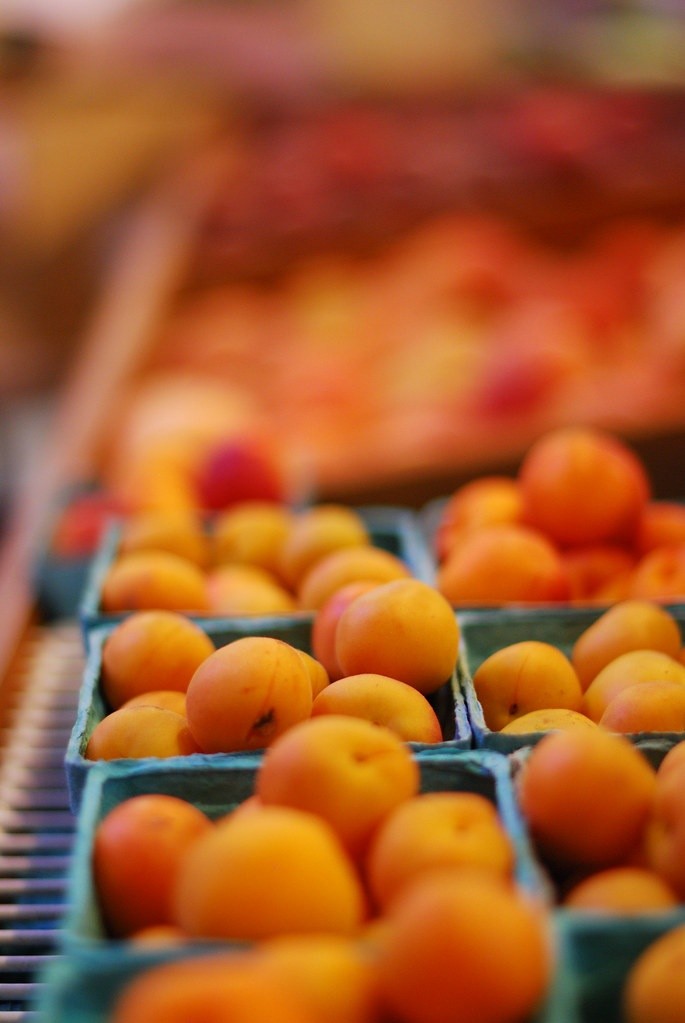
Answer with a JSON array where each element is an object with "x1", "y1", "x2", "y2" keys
[{"x1": 26, "y1": 504, "x2": 685, "y2": 1021}]
